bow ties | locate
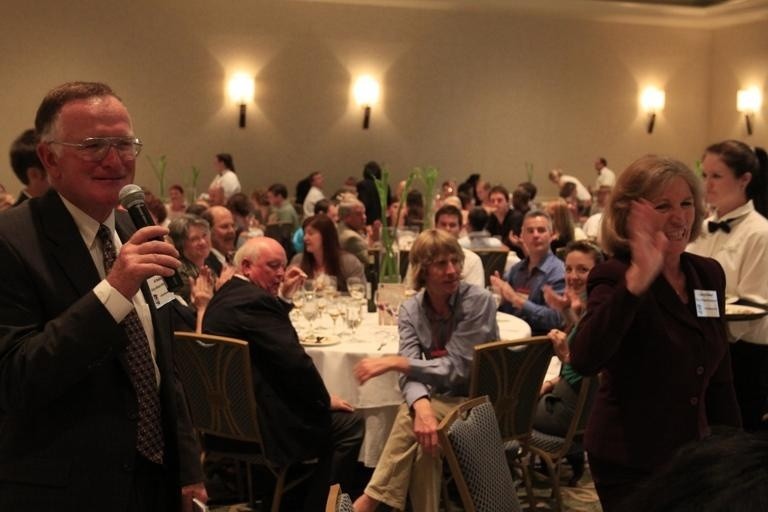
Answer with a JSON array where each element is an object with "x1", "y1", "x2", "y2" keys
[{"x1": 707, "y1": 218, "x2": 736, "y2": 234}]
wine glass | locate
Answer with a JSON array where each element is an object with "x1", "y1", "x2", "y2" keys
[
  {"x1": 373, "y1": 290, "x2": 401, "y2": 342},
  {"x1": 291, "y1": 275, "x2": 366, "y2": 341}
]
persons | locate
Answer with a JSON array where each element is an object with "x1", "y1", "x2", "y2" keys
[
  {"x1": 683, "y1": 139, "x2": 767, "y2": 436},
  {"x1": 9, "y1": 128, "x2": 51, "y2": 210},
  {"x1": 116, "y1": 151, "x2": 619, "y2": 510},
  {"x1": 567, "y1": 152, "x2": 746, "y2": 511},
  {"x1": 0, "y1": 79, "x2": 209, "y2": 511}
]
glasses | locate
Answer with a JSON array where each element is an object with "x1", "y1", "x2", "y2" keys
[{"x1": 45, "y1": 135, "x2": 143, "y2": 164}]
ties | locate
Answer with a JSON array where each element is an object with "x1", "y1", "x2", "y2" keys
[{"x1": 95, "y1": 223, "x2": 165, "y2": 465}]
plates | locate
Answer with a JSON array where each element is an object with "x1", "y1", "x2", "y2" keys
[
  {"x1": 724, "y1": 294, "x2": 766, "y2": 315},
  {"x1": 298, "y1": 337, "x2": 340, "y2": 346}
]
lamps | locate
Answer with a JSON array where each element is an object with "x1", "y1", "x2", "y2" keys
[
  {"x1": 224, "y1": 69, "x2": 257, "y2": 128},
  {"x1": 355, "y1": 76, "x2": 379, "y2": 129},
  {"x1": 734, "y1": 87, "x2": 756, "y2": 136},
  {"x1": 645, "y1": 86, "x2": 666, "y2": 134}
]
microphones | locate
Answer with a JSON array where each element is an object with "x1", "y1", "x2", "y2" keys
[{"x1": 118, "y1": 183, "x2": 184, "y2": 292}]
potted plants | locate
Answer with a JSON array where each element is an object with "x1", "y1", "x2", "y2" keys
[
  {"x1": 418, "y1": 163, "x2": 438, "y2": 230},
  {"x1": 185, "y1": 162, "x2": 203, "y2": 203},
  {"x1": 372, "y1": 168, "x2": 420, "y2": 285},
  {"x1": 145, "y1": 151, "x2": 170, "y2": 198}
]
